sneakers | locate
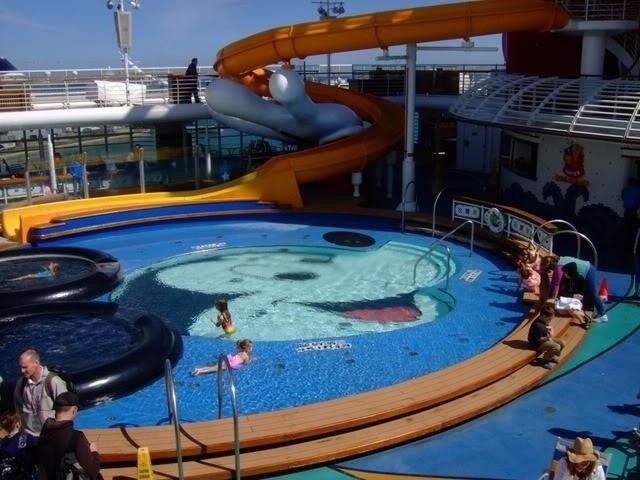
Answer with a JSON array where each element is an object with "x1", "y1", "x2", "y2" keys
[
  {"x1": 543, "y1": 355, "x2": 560, "y2": 370},
  {"x1": 592, "y1": 315, "x2": 609, "y2": 322}
]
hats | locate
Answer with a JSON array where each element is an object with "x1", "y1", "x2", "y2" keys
[
  {"x1": 54, "y1": 392, "x2": 79, "y2": 406},
  {"x1": 567, "y1": 437, "x2": 600, "y2": 464}
]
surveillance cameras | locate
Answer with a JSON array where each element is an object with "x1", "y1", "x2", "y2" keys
[
  {"x1": 130, "y1": 2, "x2": 140, "y2": 9},
  {"x1": 106, "y1": 1, "x2": 114, "y2": 9}
]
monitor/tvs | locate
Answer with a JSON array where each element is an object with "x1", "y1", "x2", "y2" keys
[{"x1": 499, "y1": 128, "x2": 541, "y2": 182}]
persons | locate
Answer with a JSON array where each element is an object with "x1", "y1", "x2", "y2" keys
[
  {"x1": 528, "y1": 307, "x2": 565, "y2": 369},
  {"x1": 553, "y1": 437, "x2": 605, "y2": 480},
  {"x1": 212, "y1": 299, "x2": 235, "y2": 340},
  {"x1": 518, "y1": 241, "x2": 609, "y2": 330},
  {"x1": 191, "y1": 339, "x2": 252, "y2": 376},
  {"x1": 4, "y1": 262, "x2": 61, "y2": 282},
  {"x1": 0, "y1": 349, "x2": 104, "y2": 480},
  {"x1": 67, "y1": 154, "x2": 84, "y2": 199},
  {"x1": 184, "y1": 58, "x2": 202, "y2": 104}
]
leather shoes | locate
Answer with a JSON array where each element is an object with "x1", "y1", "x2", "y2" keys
[{"x1": 581, "y1": 313, "x2": 591, "y2": 330}]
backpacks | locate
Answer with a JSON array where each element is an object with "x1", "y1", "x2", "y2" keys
[
  {"x1": 20, "y1": 372, "x2": 76, "y2": 401},
  {"x1": 58, "y1": 430, "x2": 89, "y2": 480}
]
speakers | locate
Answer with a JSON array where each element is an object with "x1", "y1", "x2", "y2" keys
[{"x1": 114, "y1": 11, "x2": 132, "y2": 49}]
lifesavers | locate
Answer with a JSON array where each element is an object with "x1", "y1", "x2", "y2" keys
[{"x1": 485, "y1": 208, "x2": 505, "y2": 233}]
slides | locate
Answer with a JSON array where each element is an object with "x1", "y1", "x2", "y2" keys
[{"x1": 0, "y1": 0, "x2": 569, "y2": 243}]
